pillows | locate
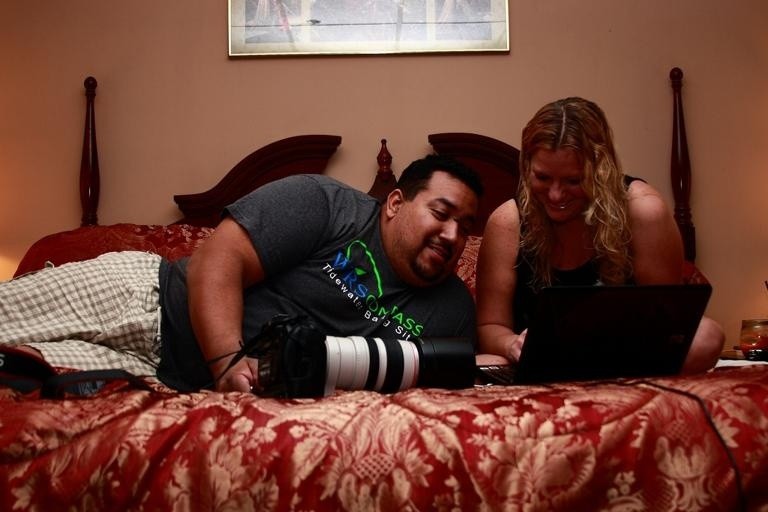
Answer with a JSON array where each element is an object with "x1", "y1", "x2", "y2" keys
[{"x1": 12, "y1": 220, "x2": 218, "y2": 280}]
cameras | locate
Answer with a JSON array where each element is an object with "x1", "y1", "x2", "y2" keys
[{"x1": 270, "y1": 313, "x2": 476, "y2": 399}]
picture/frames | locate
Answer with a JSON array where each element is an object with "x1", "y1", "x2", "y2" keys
[{"x1": 227, "y1": 0, "x2": 511, "y2": 56}]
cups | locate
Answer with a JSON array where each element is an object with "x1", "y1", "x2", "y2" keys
[{"x1": 740, "y1": 318, "x2": 768, "y2": 360}]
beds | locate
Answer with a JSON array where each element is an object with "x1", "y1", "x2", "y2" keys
[{"x1": 1, "y1": 134, "x2": 768, "y2": 511}]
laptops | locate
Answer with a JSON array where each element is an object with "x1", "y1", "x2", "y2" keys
[{"x1": 476, "y1": 284, "x2": 712, "y2": 386}]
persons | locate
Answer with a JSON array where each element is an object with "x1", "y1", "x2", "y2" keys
[
  {"x1": 0, "y1": 154, "x2": 484, "y2": 394},
  {"x1": 474, "y1": 97, "x2": 725, "y2": 386}
]
